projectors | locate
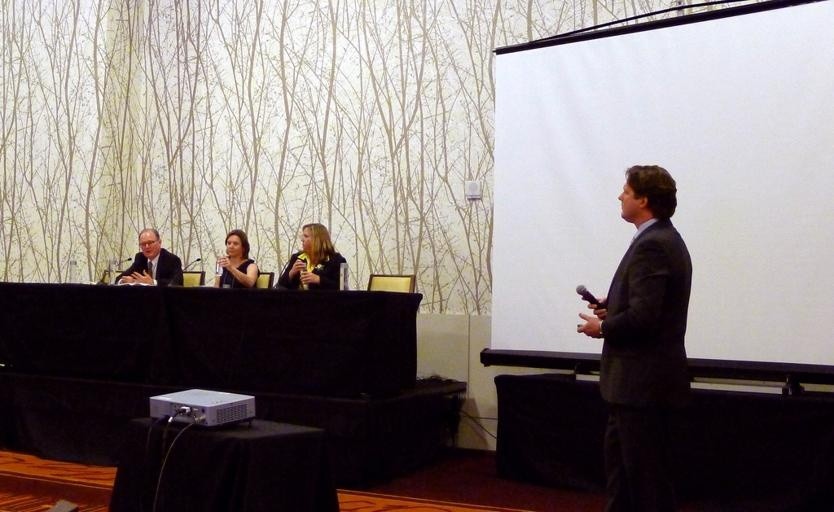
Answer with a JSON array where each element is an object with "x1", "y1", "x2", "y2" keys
[{"x1": 148, "y1": 388, "x2": 256, "y2": 428}]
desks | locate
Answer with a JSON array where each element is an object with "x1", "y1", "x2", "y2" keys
[{"x1": 1, "y1": 281, "x2": 423, "y2": 399}]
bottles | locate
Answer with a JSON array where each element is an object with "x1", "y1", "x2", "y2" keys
[
  {"x1": 215, "y1": 248, "x2": 225, "y2": 277},
  {"x1": 69, "y1": 260, "x2": 80, "y2": 283}
]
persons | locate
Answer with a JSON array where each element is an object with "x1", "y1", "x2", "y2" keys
[
  {"x1": 112, "y1": 226, "x2": 184, "y2": 286},
  {"x1": 574, "y1": 164, "x2": 693, "y2": 511},
  {"x1": 211, "y1": 229, "x2": 259, "y2": 288},
  {"x1": 277, "y1": 224, "x2": 348, "y2": 290}
]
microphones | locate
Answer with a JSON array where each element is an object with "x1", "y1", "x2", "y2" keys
[
  {"x1": 576, "y1": 284, "x2": 600, "y2": 309},
  {"x1": 169, "y1": 258, "x2": 200, "y2": 286},
  {"x1": 273, "y1": 256, "x2": 306, "y2": 289},
  {"x1": 96, "y1": 258, "x2": 132, "y2": 285}
]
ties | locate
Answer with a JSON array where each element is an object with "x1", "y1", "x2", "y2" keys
[{"x1": 148, "y1": 261, "x2": 152, "y2": 277}]
